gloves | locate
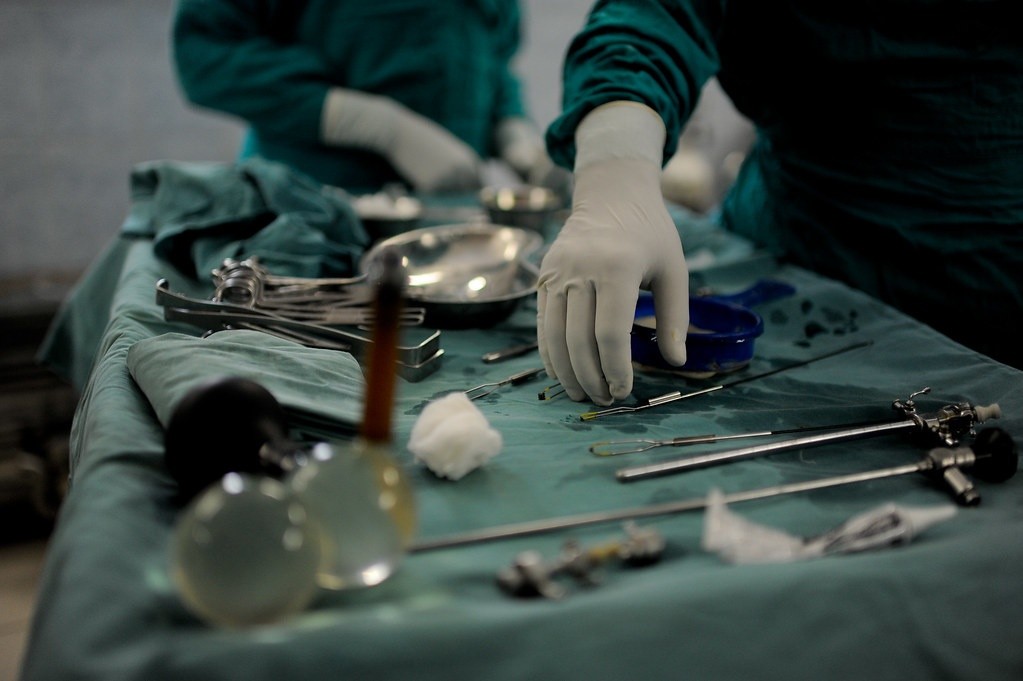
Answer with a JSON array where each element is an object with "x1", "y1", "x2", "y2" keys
[
  {"x1": 320, "y1": 85, "x2": 479, "y2": 196},
  {"x1": 496, "y1": 119, "x2": 554, "y2": 190},
  {"x1": 538, "y1": 103, "x2": 688, "y2": 410}
]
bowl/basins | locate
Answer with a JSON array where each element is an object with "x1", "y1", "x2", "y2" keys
[
  {"x1": 354, "y1": 217, "x2": 422, "y2": 243},
  {"x1": 403, "y1": 260, "x2": 539, "y2": 330},
  {"x1": 362, "y1": 223, "x2": 543, "y2": 299},
  {"x1": 479, "y1": 185, "x2": 559, "y2": 226}
]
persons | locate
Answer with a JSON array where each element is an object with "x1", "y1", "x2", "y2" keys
[
  {"x1": 536, "y1": 0, "x2": 1023, "y2": 407},
  {"x1": 172, "y1": 0, "x2": 547, "y2": 190}
]
